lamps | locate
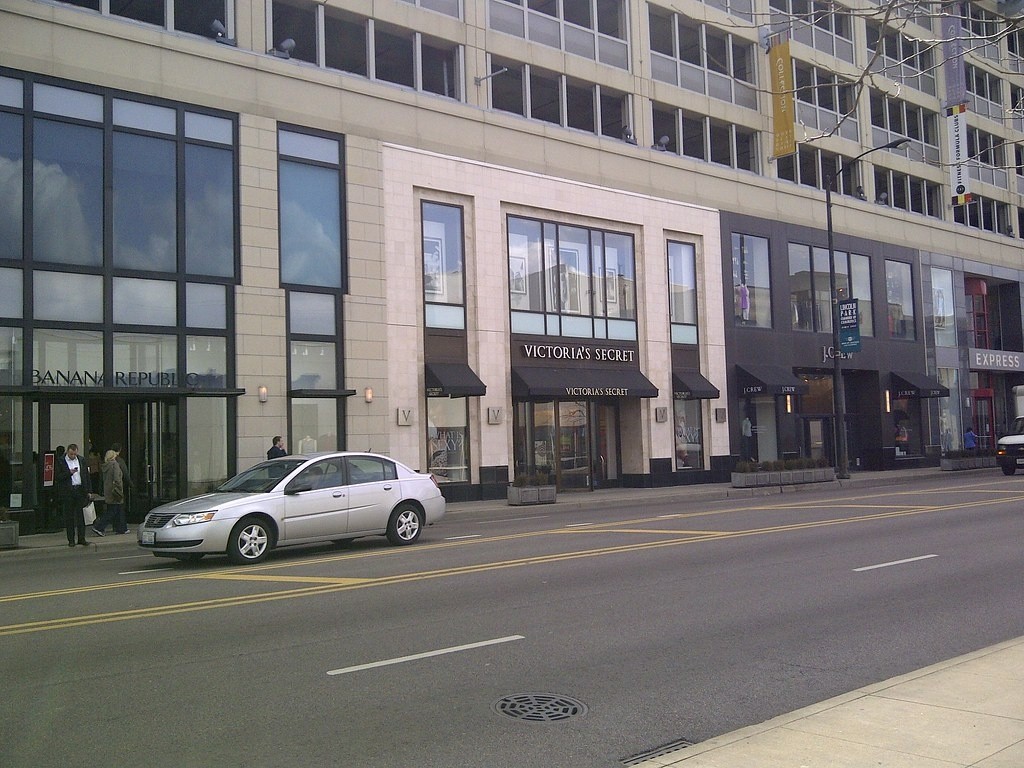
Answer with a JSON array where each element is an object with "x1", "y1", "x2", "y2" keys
[
  {"x1": 212, "y1": 18, "x2": 239, "y2": 47},
  {"x1": 621, "y1": 124, "x2": 636, "y2": 146},
  {"x1": 268, "y1": 38, "x2": 295, "y2": 60},
  {"x1": 874, "y1": 191, "x2": 888, "y2": 205},
  {"x1": 858, "y1": 185, "x2": 867, "y2": 203},
  {"x1": 651, "y1": 136, "x2": 671, "y2": 152},
  {"x1": 257, "y1": 385, "x2": 268, "y2": 403},
  {"x1": 365, "y1": 386, "x2": 373, "y2": 402}
]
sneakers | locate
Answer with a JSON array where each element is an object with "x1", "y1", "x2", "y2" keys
[
  {"x1": 92, "y1": 527, "x2": 106, "y2": 537},
  {"x1": 115, "y1": 529, "x2": 131, "y2": 534}
]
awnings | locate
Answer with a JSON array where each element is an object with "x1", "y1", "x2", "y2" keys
[
  {"x1": 672, "y1": 370, "x2": 720, "y2": 401},
  {"x1": 890, "y1": 371, "x2": 950, "y2": 400},
  {"x1": 735, "y1": 364, "x2": 809, "y2": 396},
  {"x1": 425, "y1": 363, "x2": 487, "y2": 400},
  {"x1": 511, "y1": 366, "x2": 659, "y2": 404}
]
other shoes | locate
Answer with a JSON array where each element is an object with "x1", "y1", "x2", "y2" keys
[
  {"x1": 68, "y1": 540, "x2": 75, "y2": 547},
  {"x1": 78, "y1": 540, "x2": 88, "y2": 546}
]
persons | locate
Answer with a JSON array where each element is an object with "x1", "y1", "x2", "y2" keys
[
  {"x1": 741, "y1": 417, "x2": 753, "y2": 462},
  {"x1": 31, "y1": 450, "x2": 40, "y2": 533},
  {"x1": 54, "y1": 443, "x2": 94, "y2": 547},
  {"x1": 86, "y1": 441, "x2": 108, "y2": 518},
  {"x1": 267, "y1": 435, "x2": 288, "y2": 483},
  {"x1": 308, "y1": 465, "x2": 330, "y2": 490},
  {"x1": 425, "y1": 245, "x2": 440, "y2": 291},
  {"x1": 92, "y1": 450, "x2": 131, "y2": 537},
  {"x1": 93, "y1": 442, "x2": 134, "y2": 532},
  {"x1": 318, "y1": 432, "x2": 337, "y2": 453},
  {"x1": 298, "y1": 435, "x2": 318, "y2": 455},
  {"x1": 963, "y1": 427, "x2": 978, "y2": 450},
  {"x1": 56, "y1": 445, "x2": 68, "y2": 528}
]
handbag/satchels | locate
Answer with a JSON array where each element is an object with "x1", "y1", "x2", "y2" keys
[
  {"x1": 83, "y1": 496, "x2": 97, "y2": 526},
  {"x1": 112, "y1": 487, "x2": 125, "y2": 504}
]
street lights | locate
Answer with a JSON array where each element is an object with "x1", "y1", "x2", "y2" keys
[{"x1": 824, "y1": 136, "x2": 912, "y2": 480}]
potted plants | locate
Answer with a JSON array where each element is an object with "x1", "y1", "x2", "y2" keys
[
  {"x1": 506, "y1": 469, "x2": 557, "y2": 503},
  {"x1": 939, "y1": 447, "x2": 1000, "y2": 471},
  {"x1": 0, "y1": 503, "x2": 20, "y2": 551},
  {"x1": 729, "y1": 457, "x2": 838, "y2": 488}
]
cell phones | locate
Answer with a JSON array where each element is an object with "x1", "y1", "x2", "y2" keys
[{"x1": 74, "y1": 467, "x2": 78, "y2": 470}]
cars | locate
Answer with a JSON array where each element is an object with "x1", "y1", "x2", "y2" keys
[
  {"x1": 995, "y1": 415, "x2": 1024, "y2": 475},
  {"x1": 136, "y1": 449, "x2": 449, "y2": 564}
]
